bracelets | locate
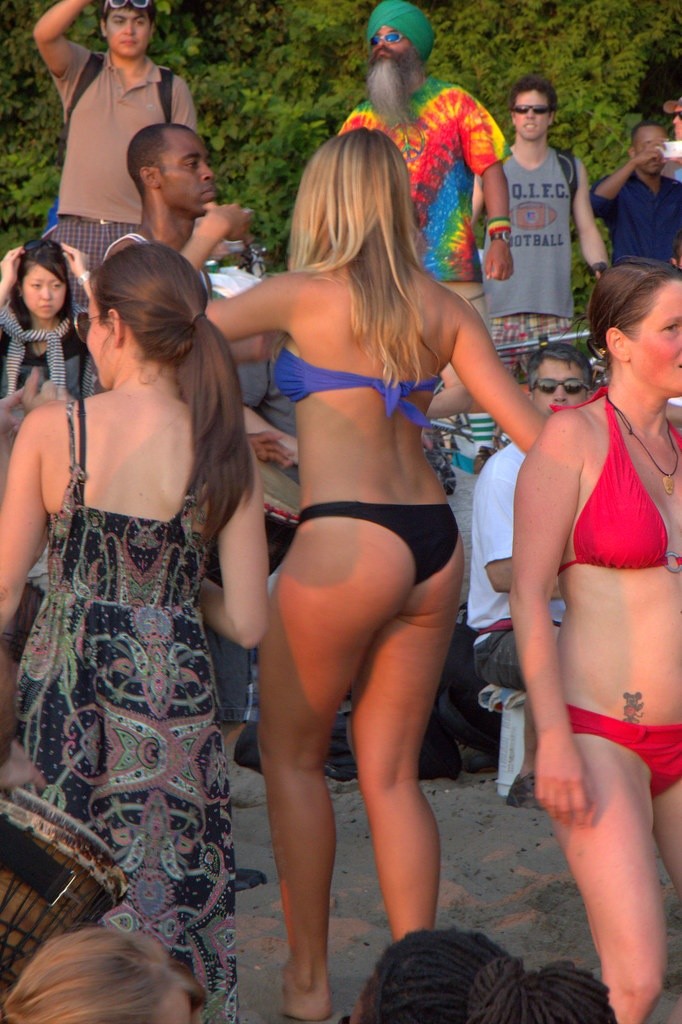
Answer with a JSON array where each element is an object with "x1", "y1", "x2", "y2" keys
[
  {"x1": 488, "y1": 217, "x2": 511, "y2": 235},
  {"x1": 78, "y1": 271, "x2": 91, "y2": 285}
]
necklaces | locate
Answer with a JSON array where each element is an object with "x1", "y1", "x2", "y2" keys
[{"x1": 606, "y1": 394, "x2": 679, "y2": 495}]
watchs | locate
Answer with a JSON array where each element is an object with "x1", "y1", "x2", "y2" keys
[{"x1": 491, "y1": 231, "x2": 511, "y2": 242}]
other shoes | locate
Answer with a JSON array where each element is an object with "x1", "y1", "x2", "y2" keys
[{"x1": 466, "y1": 753, "x2": 498, "y2": 773}]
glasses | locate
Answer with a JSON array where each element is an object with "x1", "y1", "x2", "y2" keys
[
  {"x1": 513, "y1": 104, "x2": 550, "y2": 115},
  {"x1": 370, "y1": 33, "x2": 405, "y2": 44},
  {"x1": 529, "y1": 376, "x2": 591, "y2": 395},
  {"x1": 23, "y1": 238, "x2": 61, "y2": 252},
  {"x1": 104, "y1": 0, "x2": 152, "y2": 17},
  {"x1": 673, "y1": 112, "x2": 682, "y2": 120}
]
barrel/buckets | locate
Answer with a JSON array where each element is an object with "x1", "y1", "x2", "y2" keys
[{"x1": 493, "y1": 702, "x2": 525, "y2": 796}]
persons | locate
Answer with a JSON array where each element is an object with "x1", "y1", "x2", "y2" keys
[
  {"x1": 467, "y1": 78, "x2": 607, "y2": 382},
  {"x1": 34, "y1": 0, "x2": 196, "y2": 307},
  {"x1": 591, "y1": 99, "x2": 682, "y2": 273},
  {"x1": 180, "y1": 127, "x2": 547, "y2": 1023},
  {"x1": 337, "y1": 0, "x2": 513, "y2": 334},
  {"x1": 1, "y1": 243, "x2": 269, "y2": 1024},
  {"x1": 1, "y1": 124, "x2": 472, "y2": 773},
  {"x1": 465, "y1": 345, "x2": 587, "y2": 809},
  {"x1": 350, "y1": 929, "x2": 616, "y2": 1024},
  {"x1": 510, "y1": 262, "x2": 682, "y2": 1024},
  {"x1": 0, "y1": 927, "x2": 205, "y2": 1024}
]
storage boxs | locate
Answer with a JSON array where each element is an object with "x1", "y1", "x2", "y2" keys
[{"x1": 494, "y1": 703, "x2": 526, "y2": 797}]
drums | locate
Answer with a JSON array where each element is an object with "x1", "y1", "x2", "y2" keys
[
  {"x1": 200, "y1": 458, "x2": 303, "y2": 591},
  {"x1": 0, "y1": 787, "x2": 132, "y2": 1023}
]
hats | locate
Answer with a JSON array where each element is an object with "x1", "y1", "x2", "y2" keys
[
  {"x1": 664, "y1": 95, "x2": 682, "y2": 111},
  {"x1": 367, "y1": 0, "x2": 434, "y2": 63}
]
